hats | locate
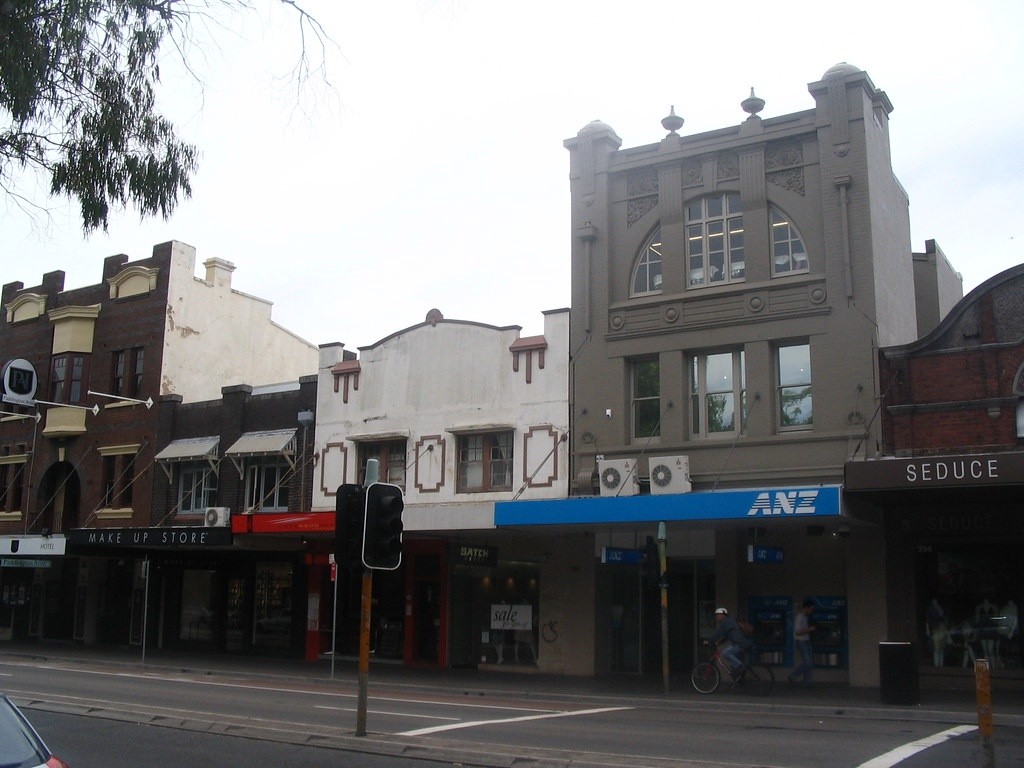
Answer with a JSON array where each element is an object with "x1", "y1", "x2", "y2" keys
[{"x1": 715, "y1": 607, "x2": 728, "y2": 616}]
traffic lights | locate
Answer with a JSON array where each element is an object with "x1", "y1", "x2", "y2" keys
[{"x1": 361, "y1": 482, "x2": 405, "y2": 570}]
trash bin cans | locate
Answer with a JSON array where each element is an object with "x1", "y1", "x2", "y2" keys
[{"x1": 878, "y1": 640, "x2": 920, "y2": 705}]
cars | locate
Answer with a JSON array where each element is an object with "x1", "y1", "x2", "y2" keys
[{"x1": 0, "y1": 693, "x2": 68, "y2": 768}]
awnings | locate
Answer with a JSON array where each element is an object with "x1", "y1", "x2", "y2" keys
[
  {"x1": 154, "y1": 436, "x2": 219, "y2": 484},
  {"x1": 226, "y1": 428, "x2": 299, "y2": 481}
]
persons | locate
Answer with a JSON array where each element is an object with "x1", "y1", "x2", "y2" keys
[
  {"x1": 786, "y1": 599, "x2": 817, "y2": 688},
  {"x1": 926, "y1": 598, "x2": 1020, "y2": 673},
  {"x1": 703, "y1": 608, "x2": 751, "y2": 694},
  {"x1": 489, "y1": 598, "x2": 538, "y2": 665}
]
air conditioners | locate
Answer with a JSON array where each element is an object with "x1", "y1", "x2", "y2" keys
[
  {"x1": 204, "y1": 507, "x2": 230, "y2": 526},
  {"x1": 648, "y1": 455, "x2": 692, "y2": 496},
  {"x1": 598, "y1": 459, "x2": 641, "y2": 496}
]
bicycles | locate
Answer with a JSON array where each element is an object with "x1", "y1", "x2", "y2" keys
[{"x1": 691, "y1": 643, "x2": 775, "y2": 698}]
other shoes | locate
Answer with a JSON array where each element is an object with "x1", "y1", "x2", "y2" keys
[
  {"x1": 787, "y1": 676, "x2": 797, "y2": 689},
  {"x1": 803, "y1": 682, "x2": 816, "y2": 689}
]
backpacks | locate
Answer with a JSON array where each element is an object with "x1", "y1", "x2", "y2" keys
[{"x1": 736, "y1": 620, "x2": 755, "y2": 635}]
